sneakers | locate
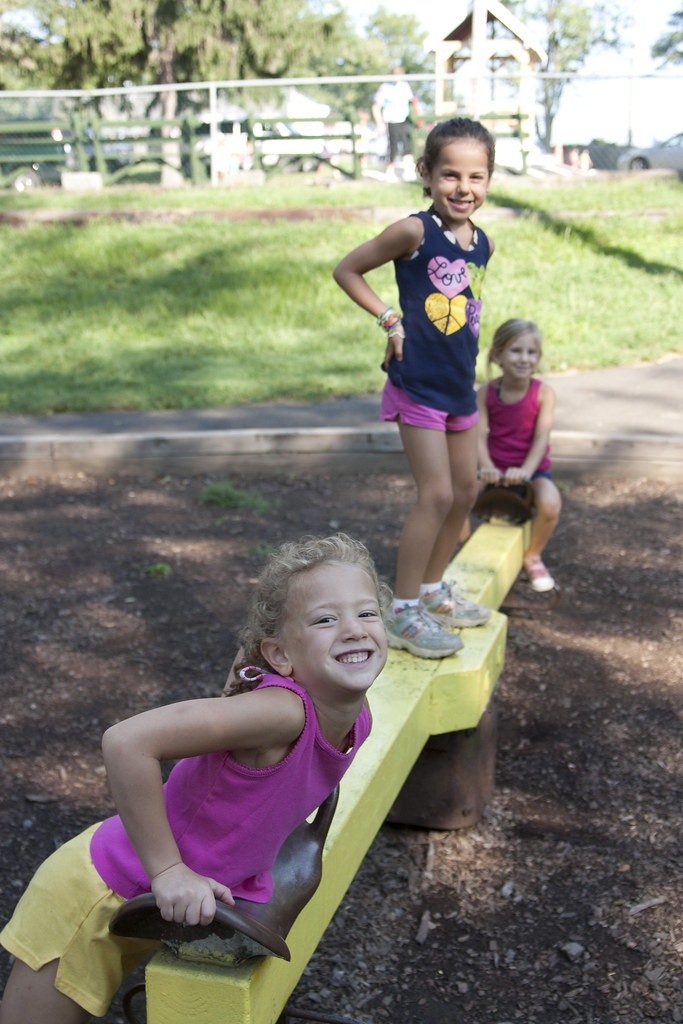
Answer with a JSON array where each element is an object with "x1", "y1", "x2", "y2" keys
[
  {"x1": 382, "y1": 603, "x2": 465, "y2": 661},
  {"x1": 521, "y1": 554, "x2": 557, "y2": 593},
  {"x1": 417, "y1": 580, "x2": 492, "y2": 631}
]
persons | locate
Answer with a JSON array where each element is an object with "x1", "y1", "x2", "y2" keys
[
  {"x1": 0, "y1": 530, "x2": 399, "y2": 1024},
  {"x1": 331, "y1": 116, "x2": 498, "y2": 663},
  {"x1": 555, "y1": 142, "x2": 594, "y2": 171},
  {"x1": 454, "y1": 316, "x2": 565, "y2": 595},
  {"x1": 373, "y1": 66, "x2": 424, "y2": 183}
]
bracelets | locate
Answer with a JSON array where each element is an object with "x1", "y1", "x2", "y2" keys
[{"x1": 376, "y1": 306, "x2": 404, "y2": 332}]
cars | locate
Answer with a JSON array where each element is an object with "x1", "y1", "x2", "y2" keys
[{"x1": 615, "y1": 131, "x2": 683, "y2": 177}]
